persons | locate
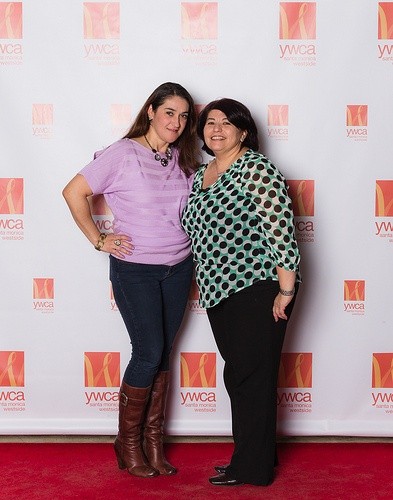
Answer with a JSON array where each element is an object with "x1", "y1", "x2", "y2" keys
[
  {"x1": 60, "y1": 80, "x2": 204, "y2": 479},
  {"x1": 179, "y1": 97, "x2": 303, "y2": 488}
]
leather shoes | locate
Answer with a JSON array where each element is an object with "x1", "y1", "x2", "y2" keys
[
  {"x1": 214, "y1": 465, "x2": 230, "y2": 474},
  {"x1": 209, "y1": 474, "x2": 245, "y2": 485}
]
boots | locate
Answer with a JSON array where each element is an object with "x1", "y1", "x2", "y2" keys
[
  {"x1": 142, "y1": 371, "x2": 177, "y2": 474},
  {"x1": 114, "y1": 379, "x2": 158, "y2": 478}
]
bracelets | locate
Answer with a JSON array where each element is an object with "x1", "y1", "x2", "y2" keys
[
  {"x1": 278, "y1": 287, "x2": 297, "y2": 297},
  {"x1": 94, "y1": 230, "x2": 107, "y2": 252}
]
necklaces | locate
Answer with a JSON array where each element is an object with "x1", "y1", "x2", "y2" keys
[
  {"x1": 213, "y1": 158, "x2": 232, "y2": 177},
  {"x1": 142, "y1": 132, "x2": 174, "y2": 167}
]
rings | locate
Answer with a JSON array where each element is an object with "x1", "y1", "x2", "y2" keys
[{"x1": 113, "y1": 238, "x2": 122, "y2": 247}]
